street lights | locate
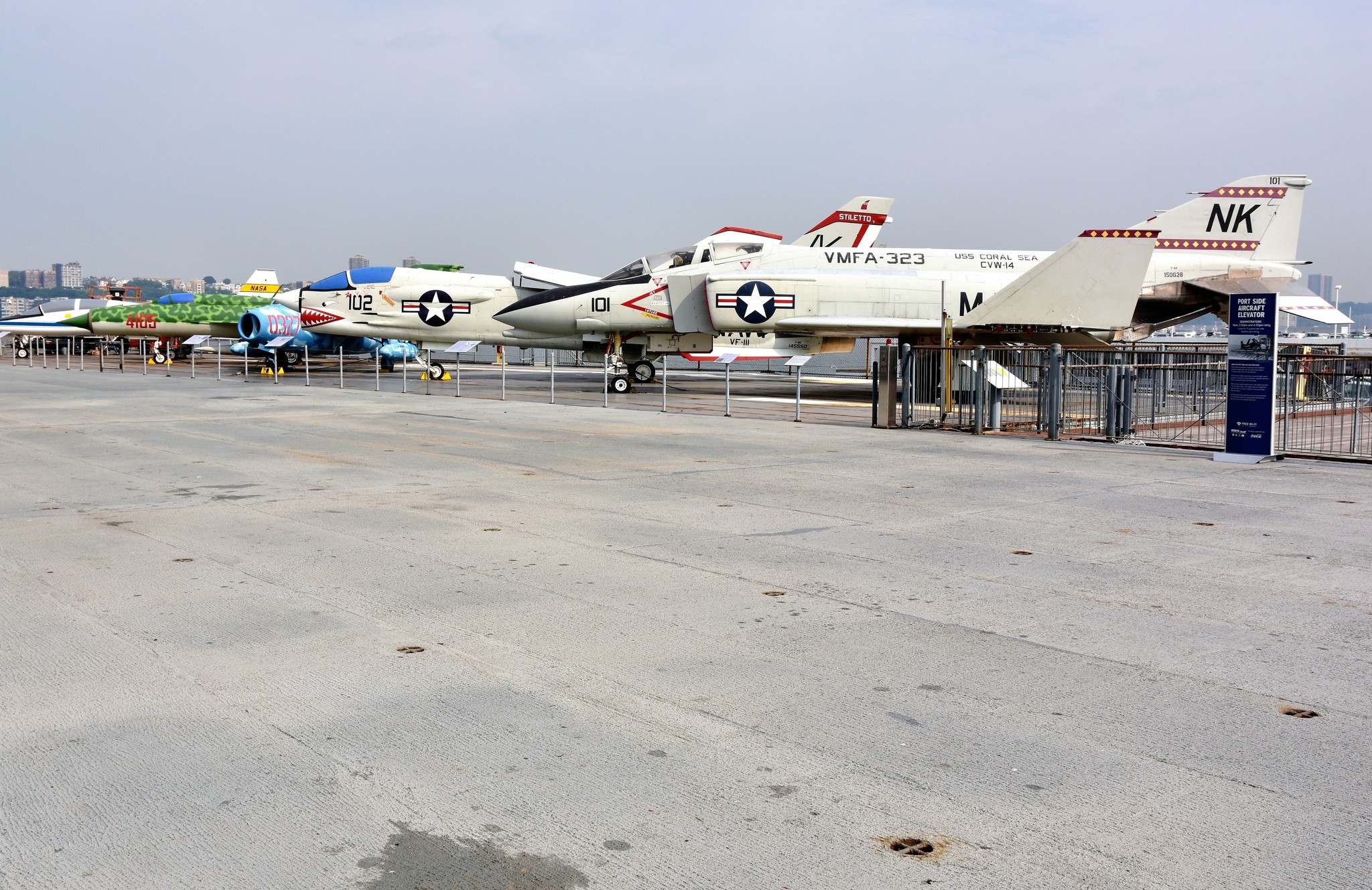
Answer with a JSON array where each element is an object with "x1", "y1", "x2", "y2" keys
[{"x1": 1334, "y1": 285, "x2": 1342, "y2": 339}]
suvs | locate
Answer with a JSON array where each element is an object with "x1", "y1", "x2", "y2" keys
[{"x1": 1278, "y1": 332, "x2": 1372, "y2": 339}]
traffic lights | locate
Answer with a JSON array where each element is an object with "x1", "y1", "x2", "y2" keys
[
  {"x1": 1366, "y1": 326, "x2": 1368, "y2": 329},
  {"x1": 1363, "y1": 327, "x2": 1364, "y2": 329}
]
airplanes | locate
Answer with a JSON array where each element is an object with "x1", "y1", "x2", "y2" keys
[{"x1": 0, "y1": 174, "x2": 1357, "y2": 394}]
